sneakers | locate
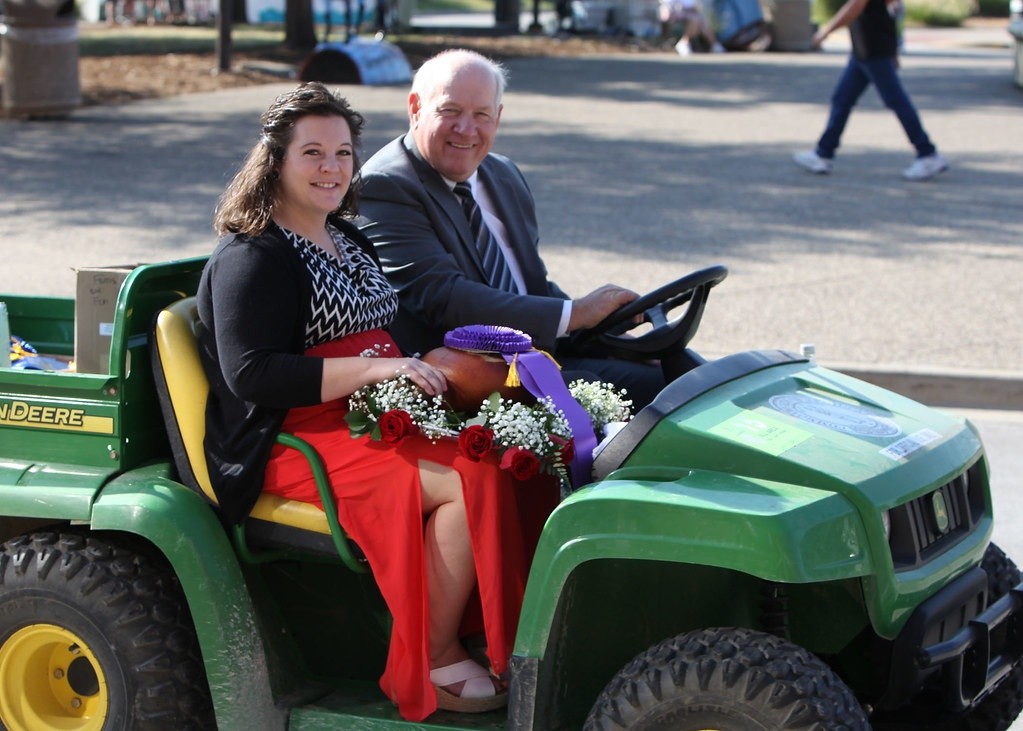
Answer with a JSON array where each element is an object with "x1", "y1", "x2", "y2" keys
[
  {"x1": 792, "y1": 150, "x2": 836, "y2": 176},
  {"x1": 903, "y1": 147, "x2": 950, "y2": 180}
]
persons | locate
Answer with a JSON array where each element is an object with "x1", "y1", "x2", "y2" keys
[
  {"x1": 675, "y1": 0, "x2": 726, "y2": 56},
  {"x1": 197, "y1": 81, "x2": 575, "y2": 721},
  {"x1": 790, "y1": 0, "x2": 950, "y2": 182},
  {"x1": 338, "y1": 47, "x2": 664, "y2": 435}
]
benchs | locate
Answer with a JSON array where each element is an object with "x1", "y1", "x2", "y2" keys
[{"x1": 149, "y1": 294, "x2": 378, "y2": 574}]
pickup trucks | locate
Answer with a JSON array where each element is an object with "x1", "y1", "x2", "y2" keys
[{"x1": 0, "y1": 253, "x2": 1022, "y2": 731}]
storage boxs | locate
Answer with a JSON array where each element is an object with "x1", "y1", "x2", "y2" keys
[{"x1": 69, "y1": 264, "x2": 153, "y2": 375}]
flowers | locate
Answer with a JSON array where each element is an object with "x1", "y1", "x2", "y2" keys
[{"x1": 344, "y1": 342, "x2": 639, "y2": 494}]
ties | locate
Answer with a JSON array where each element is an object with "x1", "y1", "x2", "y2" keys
[{"x1": 452, "y1": 180, "x2": 519, "y2": 296}]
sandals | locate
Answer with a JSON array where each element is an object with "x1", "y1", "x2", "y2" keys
[{"x1": 391, "y1": 659, "x2": 510, "y2": 714}]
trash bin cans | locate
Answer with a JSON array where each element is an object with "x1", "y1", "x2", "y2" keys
[{"x1": 0, "y1": 0, "x2": 83, "y2": 121}]
